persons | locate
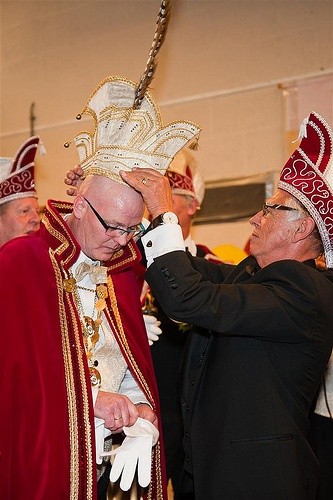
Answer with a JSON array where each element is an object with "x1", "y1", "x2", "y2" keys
[
  {"x1": 144, "y1": 148, "x2": 227, "y2": 264},
  {"x1": 0, "y1": 135, "x2": 44, "y2": 248},
  {"x1": 0, "y1": 75, "x2": 203, "y2": 500},
  {"x1": 65, "y1": 110, "x2": 333, "y2": 500}
]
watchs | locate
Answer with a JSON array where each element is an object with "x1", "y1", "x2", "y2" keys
[{"x1": 153, "y1": 211, "x2": 179, "y2": 228}]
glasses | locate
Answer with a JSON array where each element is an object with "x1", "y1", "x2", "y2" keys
[
  {"x1": 82, "y1": 196, "x2": 145, "y2": 238},
  {"x1": 262, "y1": 202, "x2": 309, "y2": 216}
]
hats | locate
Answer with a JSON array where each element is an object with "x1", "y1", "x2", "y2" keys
[
  {"x1": 164, "y1": 146, "x2": 205, "y2": 197},
  {"x1": 63, "y1": 0, "x2": 203, "y2": 201},
  {"x1": 277, "y1": 111, "x2": 333, "y2": 268},
  {"x1": 0, "y1": 136, "x2": 47, "y2": 204}
]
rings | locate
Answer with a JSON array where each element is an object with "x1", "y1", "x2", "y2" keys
[
  {"x1": 114, "y1": 416, "x2": 122, "y2": 420},
  {"x1": 142, "y1": 177, "x2": 149, "y2": 185}
]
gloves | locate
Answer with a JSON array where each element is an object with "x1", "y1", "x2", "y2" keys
[
  {"x1": 99, "y1": 417, "x2": 160, "y2": 491},
  {"x1": 94, "y1": 416, "x2": 112, "y2": 464},
  {"x1": 143, "y1": 315, "x2": 162, "y2": 346}
]
